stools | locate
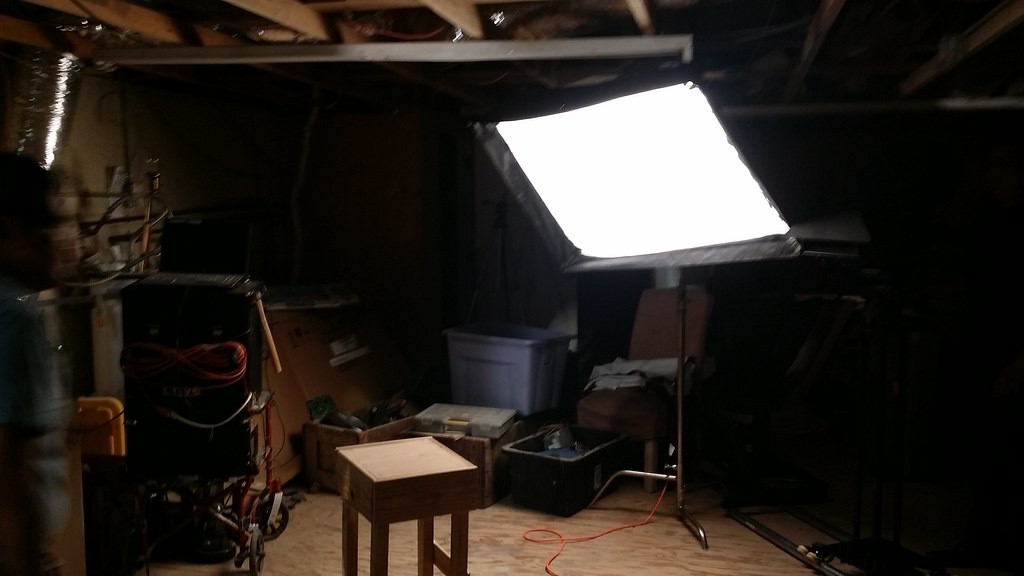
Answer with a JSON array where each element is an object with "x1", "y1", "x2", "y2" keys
[{"x1": 334, "y1": 436, "x2": 480, "y2": 576}]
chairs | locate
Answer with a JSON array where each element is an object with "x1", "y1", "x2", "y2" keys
[{"x1": 577, "y1": 285, "x2": 716, "y2": 494}]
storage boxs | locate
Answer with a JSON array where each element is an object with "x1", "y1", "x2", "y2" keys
[
  {"x1": 401, "y1": 418, "x2": 529, "y2": 510},
  {"x1": 444, "y1": 319, "x2": 572, "y2": 418},
  {"x1": 304, "y1": 416, "x2": 415, "y2": 493},
  {"x1": 501, "y1": 424, "x2": 626, "y2": 518}
]
